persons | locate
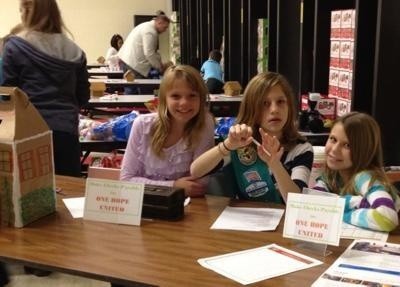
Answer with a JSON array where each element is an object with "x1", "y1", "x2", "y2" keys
[
  {"x1": 105, "y1": 15, "x2": 173, "y2": 95},
  {"x1": 200, "y1": 50, "x2": 224, "y2": 93},
  {"x1": 190, "y1": 71, "x2": 314, "y2": 206},
  {"x1": 311, "y1": 111, "x2": 400, "y2": 233},
  {"x1": 0, "y1": 0, "x2": 92, "y2": 177},
  {"x1": 119, "y1": 64, "x2": 215, "y2": 198}
]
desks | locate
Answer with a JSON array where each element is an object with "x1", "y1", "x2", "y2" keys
[
  {"x1": 81, "y1": 71, "x2": 163, "y2": 149},
  {"x1": 207, "y1": 94, "x2": 247, "y2": 102},
  {"x1": 298, "y1": 130, "x2": 331, "y2": 138},
  {"x1": 3, "y1": 175, "x2": 400, "y2": 286}
]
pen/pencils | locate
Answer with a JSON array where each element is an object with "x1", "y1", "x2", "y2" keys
[{"x1": 252, "y1": 139, "x2": 272, "y2": 158}]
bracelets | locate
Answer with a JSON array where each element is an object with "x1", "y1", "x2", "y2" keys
[
  {"x1": 222, "y1": 140, "x2": 234, "y2": 152},
  {"x1": 218, "y1": 142, "x2": 230, "y2": 156}
]
